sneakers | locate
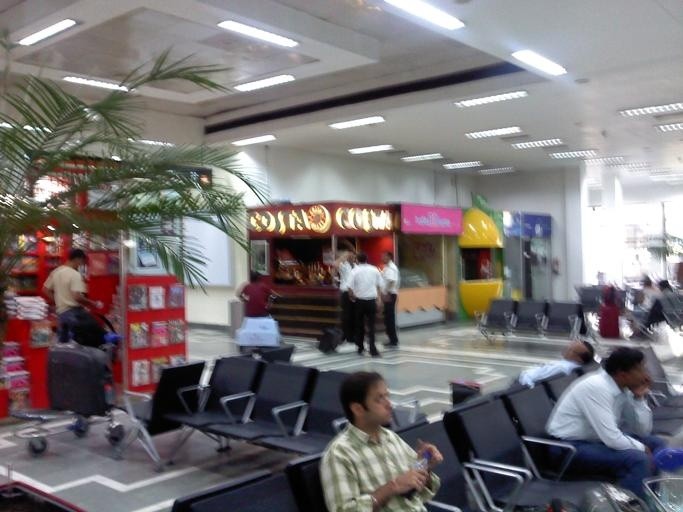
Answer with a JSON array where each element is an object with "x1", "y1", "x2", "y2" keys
[
  {"x1": 370, "y1": 350, "x2": 380, "y2": 356},
  {"x1": 384, "y1": 343, "x2": 397, "y2": 347},
  {"x1": 357, "y1": 346, "x2": 363, "y2": 353}
]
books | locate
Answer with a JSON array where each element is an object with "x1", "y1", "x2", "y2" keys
[{"x1": 1, "y1": 152, "x2": 189, "y2": 407}]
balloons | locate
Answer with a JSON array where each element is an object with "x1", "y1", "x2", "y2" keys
[{"x1": 654, "y1": 444, "x2": 683, "y2": 474}]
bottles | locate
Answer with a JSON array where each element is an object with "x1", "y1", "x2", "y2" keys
[{"x1": 408, "y1": 452, "x2": 431, "y2": 502}]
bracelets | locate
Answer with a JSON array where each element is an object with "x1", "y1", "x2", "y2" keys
[
  {"x1": 388, "y1": 480, "x2": 399, "y2": 497},
  {"x1": 371, "y1": 494, "x2": 380, "y2": 508}
]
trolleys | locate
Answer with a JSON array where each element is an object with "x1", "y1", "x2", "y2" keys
[{"x1": 12, "y1": 331, "x2": 124, "y2": 454}]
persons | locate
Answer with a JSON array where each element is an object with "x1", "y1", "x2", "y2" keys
[
  {"x1": 334, "y1": 251, "x2": 357, "y2": 342},
  {"x1": 237, "y1": 270, "x2": 283, "y2": 316},
  {"x1": 597, "y1": 252, "x2": 683, "y2": 341},
  {"x1": 347, "y1": 252, "x2": 385, "y2": 356},
  {"x1": 514, "y1": 338, "x2": 595, "y2": 388},
  {"x1": 545, "y1": 346, "x2": 669, "y2": 504},
  {"x1": 320, "y1": 371, "x2": 444, "y2": 512},
  {"x1": 375, "y1": 251, "x2": 402, "y2": 347}
]
